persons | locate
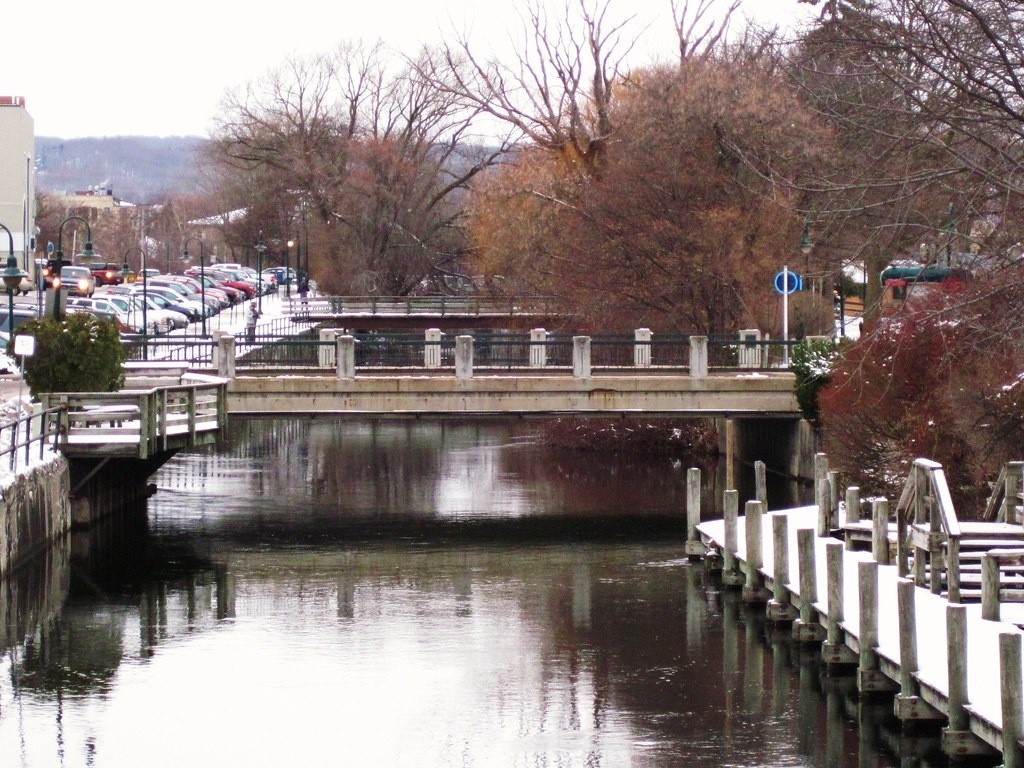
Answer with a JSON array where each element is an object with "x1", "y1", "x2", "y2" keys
[
  {"x1": 297, "y1": 273, "x2": 311, "y2": 311},
  {"x1": 833, "y1": 290, "x2": 841, "y2": 320},
  {"x1": 245, "y1": 301, "x2": 259, "y2": 343}
]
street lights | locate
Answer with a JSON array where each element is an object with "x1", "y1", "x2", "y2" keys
[
  {"x1": 288, "y1": 235, "x2": 303, "y2": 292},
  {"x1": 798, "y1": 208, "x2": 813, "y2": 285},
  {"x1": 918, "y1": 225, "x2": 954, "y2": 266},
  {"x1": 0, "y1": 222, "x2": 32, "y2": 359},
  {"x1": 183, "y1": 236, "x2": 210, "y2": 341},
  {"x1": 120, "y1": 246, "x2": 147, "y2": 361},
  {"x1": 53, "y1": 216, "x2": 93, "y2": 311},
  {"x1": 254, "y1": 228, "x2": 267, "y2": 316}
]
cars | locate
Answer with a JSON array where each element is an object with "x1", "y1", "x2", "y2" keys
[
  {"x1": 0, "y1": 263, "x2": 302, "y2": 376},
  {"x1": 0, "y1": 259, "x2": 163, "y2": 296}
]
